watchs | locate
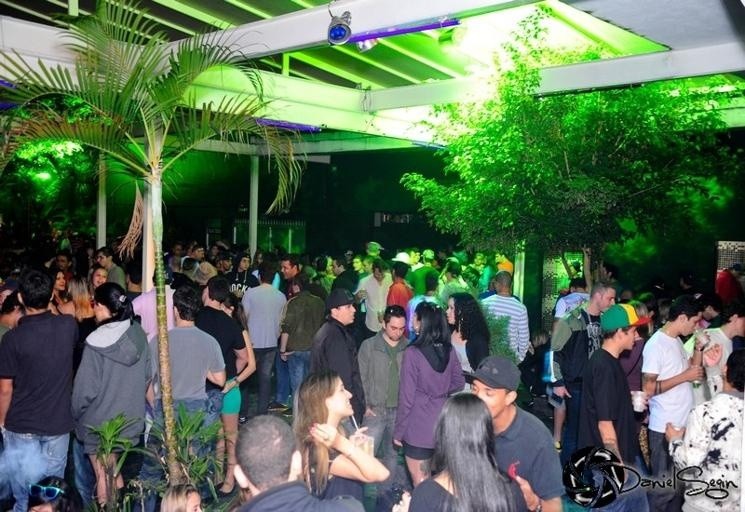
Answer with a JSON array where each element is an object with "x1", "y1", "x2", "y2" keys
[
  {"x1": 279, "y1": 351, "x2": 285, "y2": 355},
  {"x1": 536, "y1": 498, "x2": 542, "y2": 512},
  {"x1": 234, "y1": 378, "x2": 240, "y2": 386}
]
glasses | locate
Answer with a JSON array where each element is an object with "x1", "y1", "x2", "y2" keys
[
  {"x1": 386, "y1": 308, "x2": 405, "y2": 315},
  {"x1": 346, "y1": 254, "x2": 353, "y2": 257}
]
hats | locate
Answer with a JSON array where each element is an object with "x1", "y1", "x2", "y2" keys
[
  {"x1": 601, "y1": 303, "x2": 651, "y2": 331},
  {"x1": 327, "y1": 288, "x2": 356, "y2": 310},
  {"x1": 461, "y1": 356, "x2": 521, "y2": 391}
]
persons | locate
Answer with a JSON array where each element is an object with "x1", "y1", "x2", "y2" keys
[
  {"x1": 618, "y1": 316, "x2": 653, "y2": 475},
  {"x1": 232, "y1": 416, "x2": 365, "y2": 512},
  {"x1": 436, "y1": 250, "x2": 446, "y2": 260},
  {"x1": 204, "y1": 292, "x2": 257, "y2": 499},
  {"x1": 533, "y1": 332, "x2": 565, "y2": 453},
  {"x1": 353, "y1": 258, "x2": 415, "y2": 340},
  {"x1": 460, "y1": 356, "x2": 567, "y2": 512},
  {"x1": 387, "y1": 263, "x2": 412, "y2": 338},
  {"x1": 206, "y1": 241, "x2": 229, "y2": 260},
  {"x1": 96, "y1": 246, "x2": 127, "y2": 291},
  {"x1": 342, "y1": 249, "x2": 355, "y2": 270},
  {"x1": 26, "y1": 476, "x2": 84, "y2": 512},
  {"x1": 195, "y1": 274, "x2": 249, "y2": 506},
  {"x1": 411, "y1": 249, "x2": 441, "y2": 298},
  {"x1": 619, "y1": 289, "x2": 639, "y2": 308},
  {"x1": 280, "y1": 255, "x2": 303, "y2": 299},
  {"x1": 216, "y1": 255, "x2": 232, "y2": 275},
  {"x1": 462, "y1": 266, "x2": 481, "y2": 300},
  {"x1": 331, "y1": 253, "x2": 359, "y2": 295},
  {"x1": 241, "y1": 266, "x2": 287, "y2": 424},
  {"x1": 391, "y1": 392, "x2": 528, "y2": 512},
  {"x1": 44, "y1": 267, "x2": 69, "y2": 307},
  {"x1": 469, "y1": 253, "x2": 495, "y2": 291},
  {"x1": 325, "y1": 256, "x2": 338, "y2": 278},
  {"x1": 44, "y1": 248, "x2": 74, "y2": 269},
  {"x1": 406, "y1": 273, "x2": 442, "y2": 344},
  {"x1": 641, "y1": 294, "x2": 712, "y2": 512},
  {"x1": 136, "y1": 285, "x2": 226, "y2": 512},
  {"x1": 169, "y1": 258, "x2": 199, "y2": 290},
  {"x1": 553, "y1": 277, "x2": 592, "y2": 334},
  {"x1": 69, "y1": 280, "x2": 153, "y2": 512},
  {"x1": 252, "y1": 252, "x2": 279, "y2": 289},
  {"x1": 678, "y1": 272, "x2": 699, "y2": 293},
  {"x1": 309, "y1": 256, "x2": 336, "y2": 294},
  {"x1": 87, "y1": 266, "x2": 108, "y2": 297},
  {"x1": 168, "y1": 245, "x2": 184, "y2": 272},
  {"x1": 0, "y1": 267, "x2": 85, "y2": 512},
  {"x1": 305, "y1": 288, "x2": 366, "y2": 439},
  {"x1": 440, "y1": 260, "x2": 471, "y2": 300},
  {"x1": 279, "y1": 273, "x2": 327, "y2": 415},
  {"x1": 476, "y1": 271, "x2": 531, "y2": 392},
  {"x1": 392, "y1": 300, "x2": 465, "y2": 489},
  {"x1": 293, "y1": 370, "x2": 391, "y2": 512},
  {"x1": 664, "y1": 348, "x2": 744, "y2": 512},
  {"x1": 122, "y1": 262, "x2": 142, "y2": 302},
  {"x1": 682, "y1": 311, "x2": 745, "y2": 397},
  {"x1": 582, "y1": 303, "x2": 652, "y2": 512},
  {"x1": 597, "y1": 261, "x2": 622, "y2": 298},
  {"x1": 180, "y1": 240, "x2": 203, "y2": 270},
  {"x1": 196, "y1": 261, "x2": 216, "y2": 285},
  {"x1": 549, "y1": 280, "x2": 616, "y2": 453},
  {"x1": 358, "y1": 305, "x2": 412, "y2": 498},
  {"x1": 445, "y1": 291, "x2": 493, "y2": 393},
  {"x1": 714, "y1": 264, "x2": 743, "y2": 311},
  {"x1": 180, "y1": 245, "x2": 204, "y2": 271},
  {"x1": 160, "y1": 483, "x2": 202, "y2": 512},
  {"x1": 301, "y1": 257, "x2": 318, "y2": 281},
  {"x1": 494, "y1": 247, "x2": 514, "y2": 276},
  {"x1": 227, "y1": 254, "x2": 260, "y2": 302},
  {"x1": 132, "y1": 265, "x2": 177, "y2": 344},
  {"x1": 56, "y1": 277, "x2": 99, "y2": 375}
]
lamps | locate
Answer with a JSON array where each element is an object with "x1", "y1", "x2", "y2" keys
[{"x1": 327, "y1": 10, "x2": 352, "y2": 46}]
